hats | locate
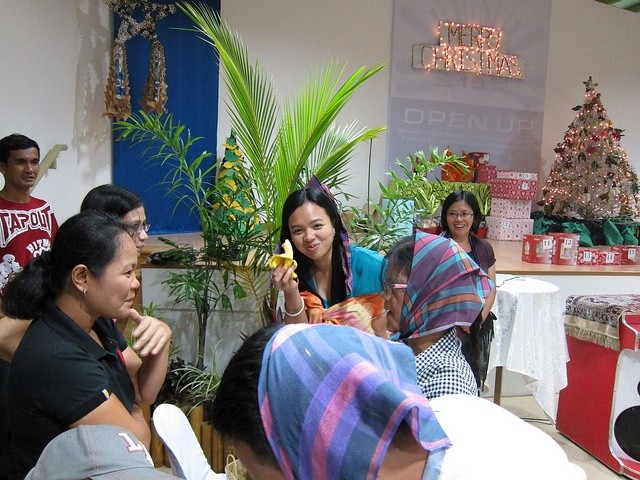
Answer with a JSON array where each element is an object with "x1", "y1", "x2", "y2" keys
[{"x1": 24, "y1": 424, "x2": 184, "y2": 480}]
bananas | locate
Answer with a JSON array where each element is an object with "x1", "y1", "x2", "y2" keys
[{"x1": 269, "y1": 240, "x2": 298, "y2": 278}]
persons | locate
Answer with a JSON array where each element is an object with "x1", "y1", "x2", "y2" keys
[
  {"x1": 1, "y1": 210, "x2": 172, "y2": 480},
  {"x1": 269, "y1": 189, "x2": 389, "y2": 339},
  {"x1": 379, "y1": 229, "x2": 493, "y2": 398},
  {"x1": 1, "y1": 133, "x2": 60, "y2": 293},
  {"x1": 435, "y1": 191, "x2": 496, "y2": 396},
  {"x1": 0, "y1": 184, "x2": 152, "y2": 366},
  {"x1": 211, "y1": 323, "x2": 586, "y2": 480}
]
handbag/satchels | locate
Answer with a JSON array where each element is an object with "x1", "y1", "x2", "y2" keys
[{"x1": 225, "y1": 454, "x2": 247, "y2": 480}]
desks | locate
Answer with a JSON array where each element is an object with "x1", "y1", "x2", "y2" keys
[{"x1": 491, "y1": 273, "x2": 571, "y2": 429}]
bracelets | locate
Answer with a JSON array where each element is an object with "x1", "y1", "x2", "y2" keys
[{"x1": 283, "y1": 295, "x2": 304, "y2": 317}]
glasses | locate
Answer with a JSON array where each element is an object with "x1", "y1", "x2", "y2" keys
[
  {"x1": 133, "y1": 224, "x2": 150, "y2": 234},
  {"x1": 446, "y1": 211, "x2": 474, "y2": 219},
  {"x1": 384, "y1": 281, "x2": 407, "y2": 290}
]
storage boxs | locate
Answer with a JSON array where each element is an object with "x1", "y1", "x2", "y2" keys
[
  {"x1": 434, "y1": 181, "x2": 491, "y2": 215},
  {"x1": 468, "y1": 151, "x2": 493, "y2": 165},
  {"x1": 613, "y1": 244, "x2": 639, "y2": 265},
  {"x1": 381, "y1": 198, "x2": 412, "y2": 234},
  {"x1": 474, "y1": 165, "x2": 495, "y2": 183},
  {"x1": 489, "y1": 178, "x2": 537, "y2": 198},
  {"x1": 497, "y1": 171, "x2": 538, "y2": 182},
  {"x1": 438, "y1": 156, "x2": 475, "y2": 182},
  {"x1": 489, "y1": 197, "x2": 533, "y2": 219},
  {"x1": 599, "y1": 252, "x2": 623, "y2": 267},
  {"x1": 576, "y1": 248, "x2": 599, "y2": 266},
  {"x1": 484, "y1": 216, "x2": 534, "y2": 241},
  {"x1": 521, "y1": 233, "x2": 554, "y2": 264},
  {"x1": 547, "y1": 231, "x2": 580, "y2": 266}
]
